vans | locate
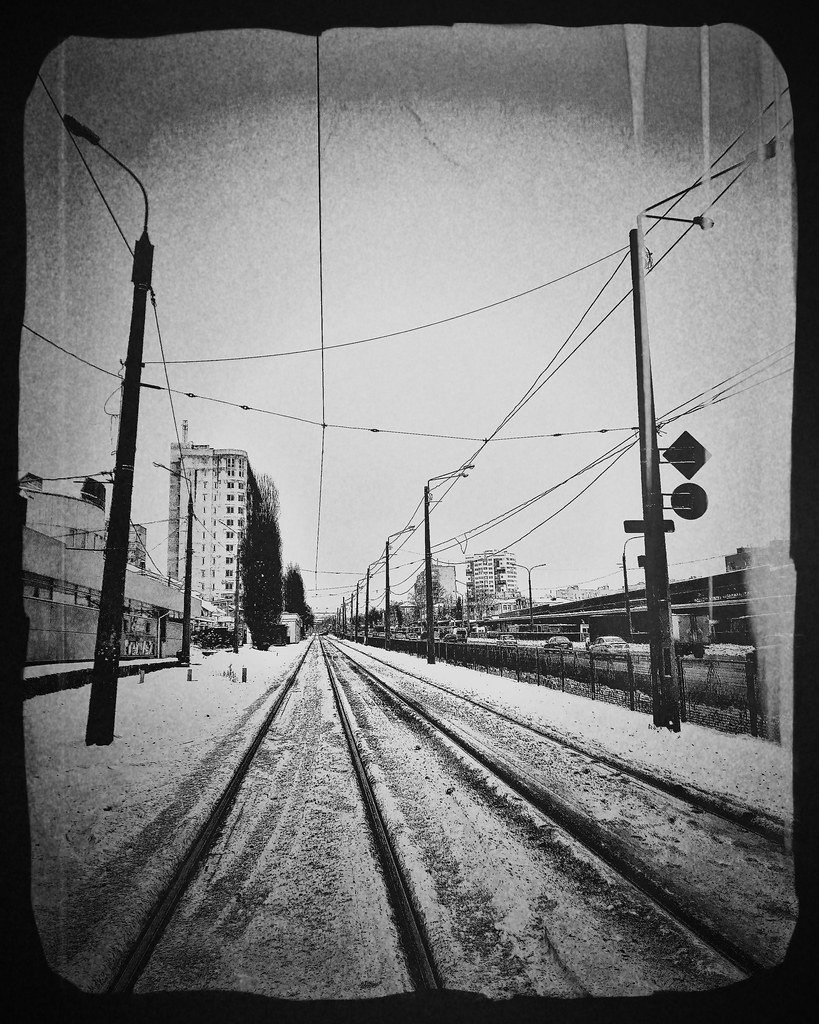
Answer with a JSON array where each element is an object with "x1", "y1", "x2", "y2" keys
[{"x1": 453, "y1": 627, "x2": 468, "y2": 644}]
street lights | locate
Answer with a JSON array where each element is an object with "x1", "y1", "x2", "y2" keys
[
  {"x1": 420, "y1": 461, "x2": 475, "y2": 666},
  {"x1": 60, "y1": 108, "x2": 156, "y2": 747},
  {"x1": 646, "y1": 135, "x2": 779, "y2": 730},
  {"x1": 383, "y1": 524, "x2": 416, "y2": 651},
  {"x1": 364, "y1": 552, "x2": 398, "y2": 647},
  {"x1": 511, "y1": 562, "x2": 547, "y2": 630}
]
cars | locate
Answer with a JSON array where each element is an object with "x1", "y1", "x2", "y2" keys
[
  {"x1": 496, "y1": 634, "x2": 517, "y2": 648},
  {"x1": 543, "y1": 636, "x2": 574, "y2": 654},
  {"x1": 443, "y1": 633, "x2": 457, "y2": 644},
  {"x1": 405, "y1": 633, "x2": 418, "y2": 641},
  {"x1": 589, "y1": 636, "x2": 630, "y2": 658}
]
